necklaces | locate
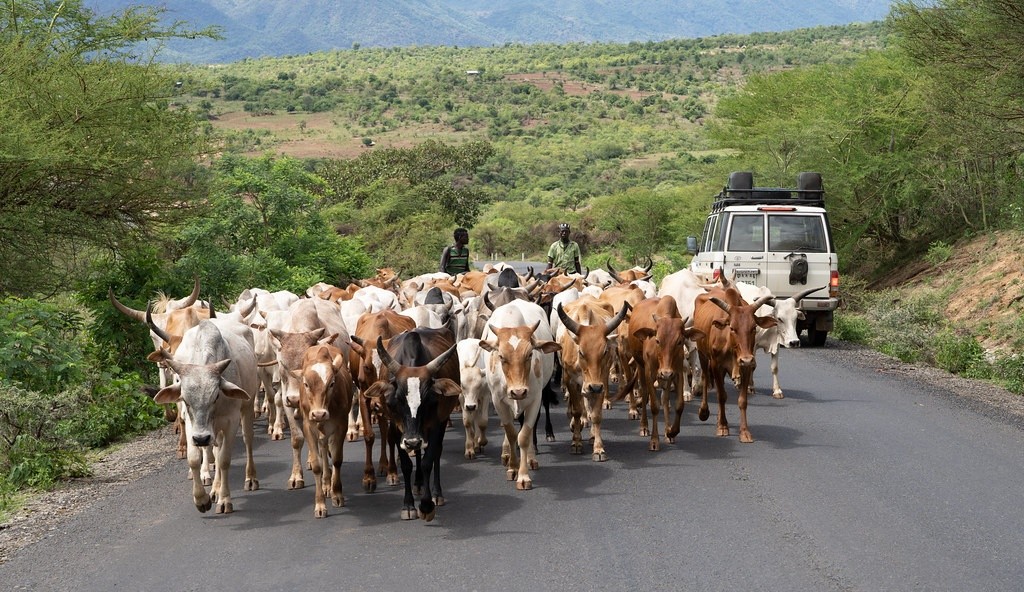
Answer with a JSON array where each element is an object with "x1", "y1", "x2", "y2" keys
[{"x1": 559, "y1": 241, "x2": 568, "y2": 248}]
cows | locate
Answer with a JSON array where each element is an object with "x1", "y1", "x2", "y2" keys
[{"x1": 109, "y1": 255, "x2": 827, "y2": 523}]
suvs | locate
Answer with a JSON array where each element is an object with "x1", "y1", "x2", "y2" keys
[{"x1": 686, "y1": 171, "x2": 839, "y2": 344}]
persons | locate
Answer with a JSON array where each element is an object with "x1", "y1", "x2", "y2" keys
[
  {"x1": 546, "y1": 223, "x2": 582, "y2": 274},
  {"x1": 440, "y1": 229, "x2": 470, "y2": 276}
]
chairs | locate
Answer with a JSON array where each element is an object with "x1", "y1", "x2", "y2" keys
[{"x1": 775, "y1": 223, "x2": 809, "y2": 250}]
